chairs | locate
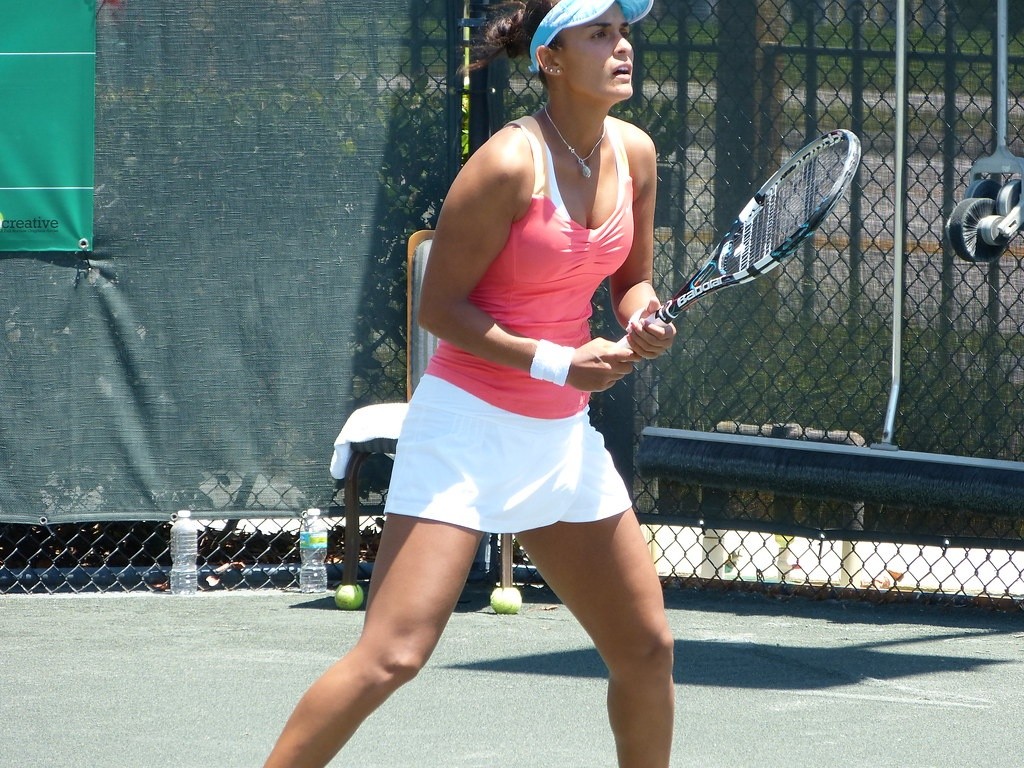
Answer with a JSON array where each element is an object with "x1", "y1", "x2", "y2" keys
[{"x1": 342, "y1": 230, "x2": 514, "y2": 614}]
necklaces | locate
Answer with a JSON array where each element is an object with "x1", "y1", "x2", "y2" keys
[{"x1": 542, "y1": 105, "x2": 606, "y2": 178}]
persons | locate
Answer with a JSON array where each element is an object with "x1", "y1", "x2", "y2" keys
[{"x1": 258, "y1": 1, "x2": 675, "y2": 764}]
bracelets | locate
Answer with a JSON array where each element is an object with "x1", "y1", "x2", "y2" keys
[{"x1": 528, "y1": 339, "x2": 574, "y2": 387}]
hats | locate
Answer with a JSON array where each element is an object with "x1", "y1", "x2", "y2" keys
[{"x1": 528, "y1": 0, "x2": 654, "y2": 74}]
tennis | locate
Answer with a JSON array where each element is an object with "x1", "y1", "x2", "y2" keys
[
  {"x1": 333, "y1": 583, "x2": 366, "y2": 611},
  {"x1": 491, "y1": 586, "x2": 523, "y2": 615}
]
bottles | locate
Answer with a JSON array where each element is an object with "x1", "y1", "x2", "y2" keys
[
  {"x1": 299, "y1": 508, "x2": 328, "y2": 594},
  {"x1": 170, "y1": 510, "x2": 198, "y2": 596}
]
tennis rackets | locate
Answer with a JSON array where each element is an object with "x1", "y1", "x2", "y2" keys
[{"x1": 613, "y1": 128, "x2": 862, "y2": 351}]
